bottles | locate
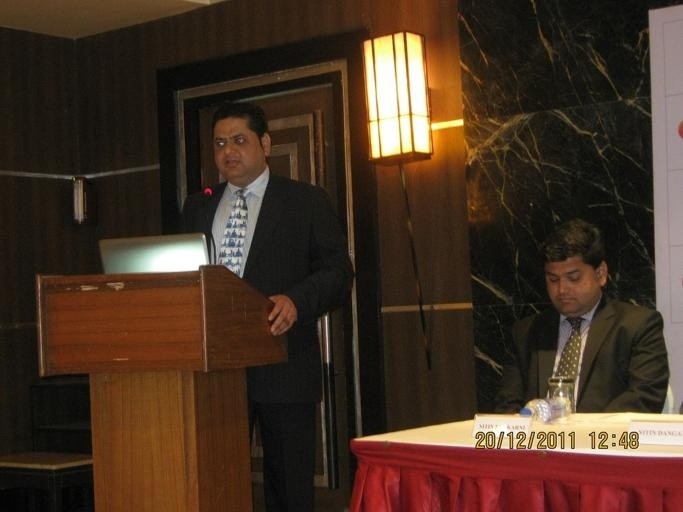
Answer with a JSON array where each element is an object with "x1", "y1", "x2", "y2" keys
[{"x1": 519, "y1": 397, "x2": 571, "y2": 423}]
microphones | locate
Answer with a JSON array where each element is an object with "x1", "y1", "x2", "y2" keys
[{"x1": 203, "y1": 183, "x2": 217, "y2": 265}]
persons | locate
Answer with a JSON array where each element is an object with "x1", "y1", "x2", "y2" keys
[
  {"x1": 492, "y1": 217, "x2": 669, "y2": 419},
  {"x1": 171, "y1": 101, "x2": 354, "y2": 510}
]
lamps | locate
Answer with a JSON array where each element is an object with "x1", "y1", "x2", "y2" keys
[{"x1": 361, "y1": 32, "x2": 433, "y2": 372}]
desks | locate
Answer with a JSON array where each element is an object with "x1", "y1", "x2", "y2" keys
[{"x1": 350, "y1": 413, "x2": 683, "y2": 512}]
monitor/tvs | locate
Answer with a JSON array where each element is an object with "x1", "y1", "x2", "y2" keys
[{"x1": 99, "y1": 233, "x2": 210, "y2": 274}]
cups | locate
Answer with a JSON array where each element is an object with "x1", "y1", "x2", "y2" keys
[{"x1": 547, "y1": 374, "x2": 575, "y2": 413}]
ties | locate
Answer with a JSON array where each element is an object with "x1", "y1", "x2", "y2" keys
[
  {"x1": 551, "y1": 314, "x2": 585, "y2": 411},
  {"x1": 216, "y1": 189, "x2": 254, "y2": 278}
]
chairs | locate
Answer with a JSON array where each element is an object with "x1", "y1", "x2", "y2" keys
[{"x1": 0, "y1": 380, "x2": 94, "y2": 512}]
